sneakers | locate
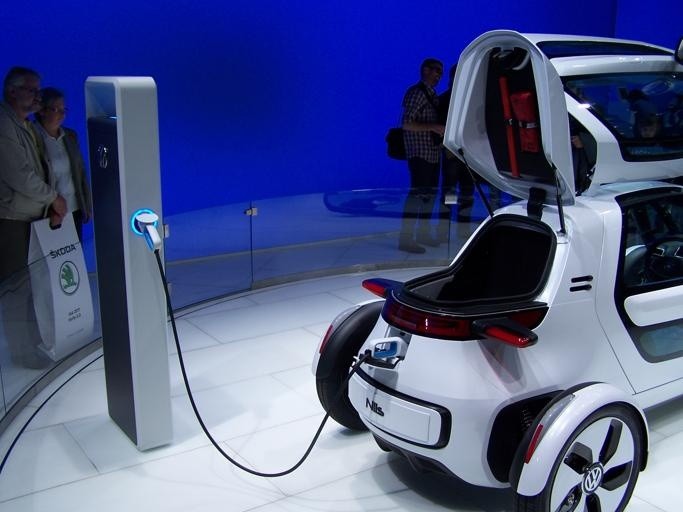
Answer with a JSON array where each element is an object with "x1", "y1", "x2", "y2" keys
[
  {"x1": 416, "y1": 232, "x2": 442, "y2": 248},
  {"x1": 398, "y1": 236, "x2": 425, "y2": 253},
  {"x1": 9, "y1": 352, "x2": 47, "y2": 371}
]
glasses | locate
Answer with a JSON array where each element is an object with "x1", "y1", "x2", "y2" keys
[{"x1": 18, "y1": 85, "x2": 44, "y2": 98}]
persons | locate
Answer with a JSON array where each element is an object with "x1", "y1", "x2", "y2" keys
[
  {"x1": 437, "y1": 64, "x2": 477, "y2": 243},
  {"x1": 34, "y1": 85, "x2": 91, "y2": 250},
  {"x1": 0, "y1": 66, "x2": 68, "y2": 371},
  {"x1": 394, "y1": 59, "x2": 442, "y2": 255}
]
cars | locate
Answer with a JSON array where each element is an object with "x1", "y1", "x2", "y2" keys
[{"x1": 316, "y1": 29, "x2": 683, "y2": 512}]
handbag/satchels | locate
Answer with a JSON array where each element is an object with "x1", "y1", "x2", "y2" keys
[
  {"x1": 383, "y1": 126, "x2": 410, "y2": 159},
  {"x1": 27, "y1": 209, "x2": 96, "y2": 358}
]
